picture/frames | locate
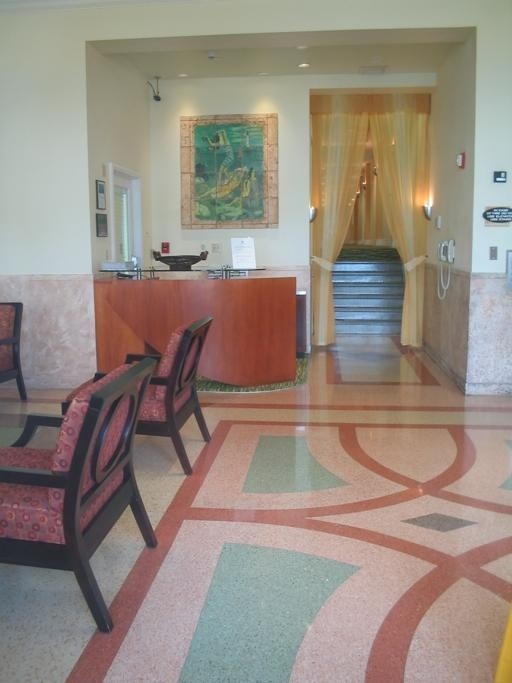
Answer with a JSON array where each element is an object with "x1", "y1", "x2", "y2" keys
[{"x1": 96, "y1": 179, "x2": 109, "y2": 237}]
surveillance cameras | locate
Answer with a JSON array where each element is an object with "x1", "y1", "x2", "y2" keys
[{"x1": 154, "y1": 95, "x2": 160, "y2": 101}]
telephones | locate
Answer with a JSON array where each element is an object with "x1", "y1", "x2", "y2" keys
[{"x1": 437, "y1": 239, "x2": 455, "y2": 264}]
